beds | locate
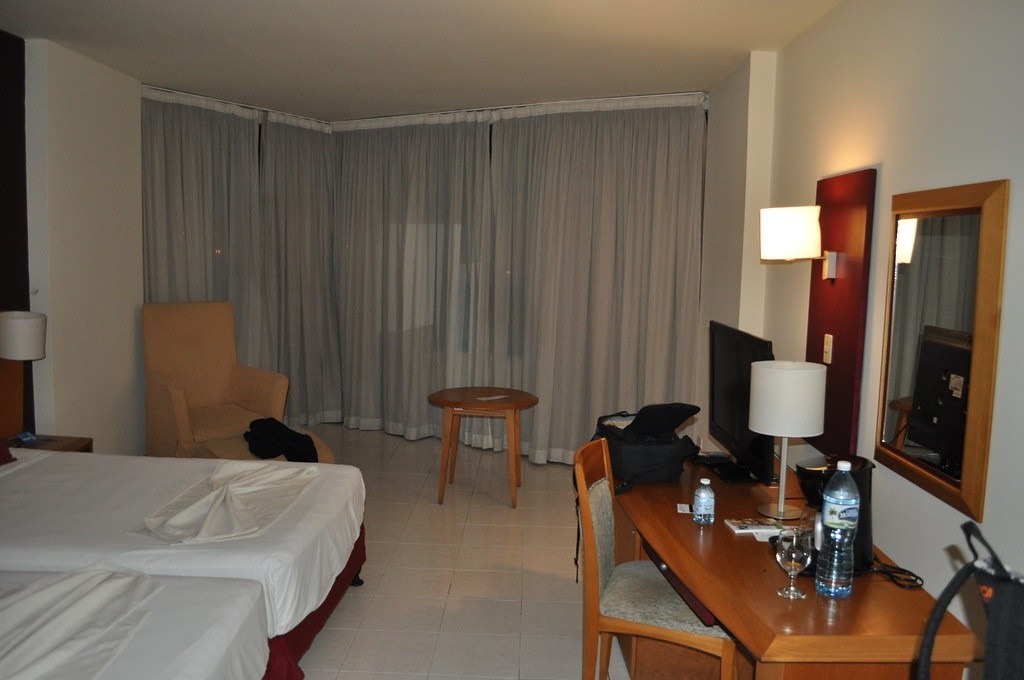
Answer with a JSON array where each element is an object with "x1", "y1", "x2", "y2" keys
[{"x1": 0, "y1": 359, "x2": 365, "y2": 680}]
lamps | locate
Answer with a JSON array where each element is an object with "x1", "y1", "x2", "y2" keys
[
  {"x1": 760, "y1": 206, "x2": 836, "y2": 280},
  {"x1": 0, "y1": 310, "x2": 47, "y2": 361},
  {"x1": 748, "y1": 360, "x2": 827, "y2": 521}
]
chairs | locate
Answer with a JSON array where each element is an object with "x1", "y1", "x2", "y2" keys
[
  {"x1": 141, "y1": 300, "x2": 289, "y2": 460},
  {"x1": 574, "y1": 437, "x2": 739, "y2": 680}
]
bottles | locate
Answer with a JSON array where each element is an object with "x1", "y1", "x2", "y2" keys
[
  {"x1": 694, "y1": 478, "x2": 715, "y2": 524},
  {"x1": 815, "y1": 461, "x2": 860, "y2": 598}
]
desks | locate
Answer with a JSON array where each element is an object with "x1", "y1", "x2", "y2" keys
[
  {"x1": 605, "y1": 454, "x2": 987, "y2": 680},
  {"x1": 426, "y1": 385, "x2": 539, "y2": 508},
  {"x1": 888, "y1": 396, "x2": 912, "y2": 450}
]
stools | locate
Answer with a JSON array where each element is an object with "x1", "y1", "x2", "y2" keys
[{"x1": 188, "y1": 430, "x2": 335, "y2": 472}]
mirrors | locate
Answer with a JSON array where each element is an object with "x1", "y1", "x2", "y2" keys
[{"x1": 874, "y1": 180, "x2": 1010, "y2": 523}]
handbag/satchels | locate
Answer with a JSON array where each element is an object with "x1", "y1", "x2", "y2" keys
[
  {"x1": 959, "y1": 521, "x2": 1024, "y2": 679},
  {"x1": 586, "y1": 400, "x2": 700, "y2": 493}
]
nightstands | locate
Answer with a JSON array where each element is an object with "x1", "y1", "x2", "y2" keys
[{"x1": 11, "y1": 434, "x2": 93, "y2": 453}]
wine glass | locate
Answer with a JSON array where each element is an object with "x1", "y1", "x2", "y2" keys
[{"x1": 777, "y1": 530, "x2": 812, "y2": 600}]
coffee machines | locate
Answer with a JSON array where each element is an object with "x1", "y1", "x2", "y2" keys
[{"x1": 791, "y1": 455, "x2": 876, "y2": 581}]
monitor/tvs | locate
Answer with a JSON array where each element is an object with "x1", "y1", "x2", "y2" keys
[
  {"x1": 907, "y1": 325, "x2": 974, "y2": 480},
  {"x1": 700, "y1": 320, "x2": 779, "y2": 488}
]
